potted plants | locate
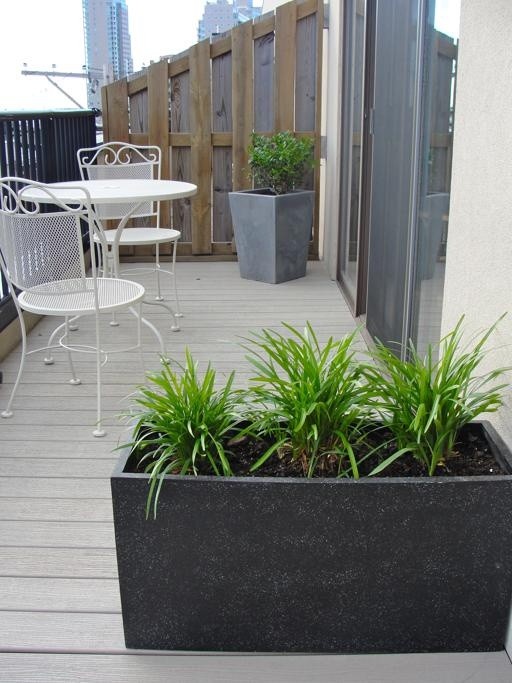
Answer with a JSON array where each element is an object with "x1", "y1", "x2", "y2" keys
[
  {"x1": 226, "y1": 131, "x2": 317, "y2": 288},
  {"x1": 105, "y1": 308, "x2": 512, "y2": 655}
]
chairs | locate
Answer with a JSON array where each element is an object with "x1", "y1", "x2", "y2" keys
[
  {"x1": 1, "y1": 175, "x2": 146, "y2": 438},
  {"x1": 76, "y1": 137, "x2": 166, "y2": 303}
]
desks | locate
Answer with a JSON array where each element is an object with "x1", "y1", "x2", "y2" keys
[{"x1": 19, "y1": 179, "x2": 198, "y2": 370}]
